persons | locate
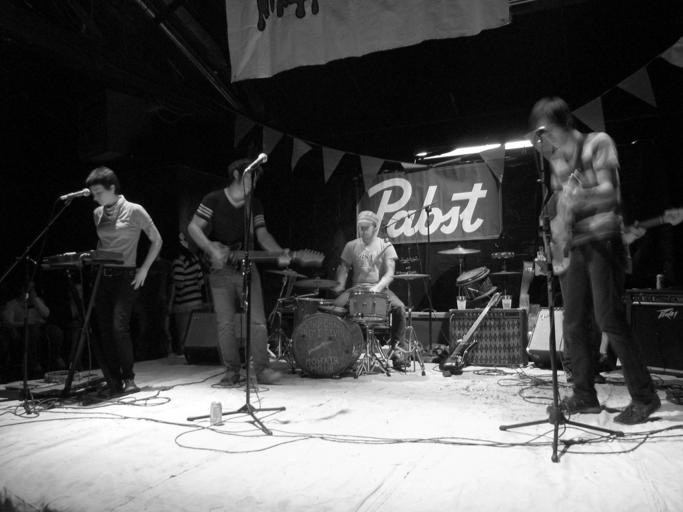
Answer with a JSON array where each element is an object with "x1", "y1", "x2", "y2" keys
[
  {"x1": 0, "y1": 229, "x2": 205, "y2": 383},
  {"x1": 325, "y1": 211, "x2": 413, "y2": 371},
  {"x1": 78, "y1": 167, "x2": 167, "y2": 406},
  {"x1": 186, "y1": 154, "x2": 292, "y2": 387},
  {"x1": 527, "y1": 92, "x2": 662, "y2": 424}
]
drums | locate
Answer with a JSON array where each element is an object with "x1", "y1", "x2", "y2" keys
[
  {"x1": 364, "y1": 302, "x2": 393, "y2": 330},
  {"x1": 456, "y1": 266, "x2": 498, "y2": 305},
  {"x1": 348, "y1": 290, "x2": 389, "y2": 322},
  {"x1": 291, "y1": 314, "x2": 363, "y2": 376},
  {"x1": 292, "y1": 297, "x2": 335, "y2": 329},
  {"x1": 315, "y1": 304, "x2": 346, "y2": 318}
]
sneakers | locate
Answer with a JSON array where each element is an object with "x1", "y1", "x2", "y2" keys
[
  {"x1": 220, "y1": 368, "x2": 241, "y2": 386},
  {"x1": 615, "y1": 390, "x2": 662, "y2": 425},
  {"x1": 546, "y1": 390, "x2": 602, "y2": 415},
  {"x1": 256, "y1": 366, "x2": 281, "y2": 384}
]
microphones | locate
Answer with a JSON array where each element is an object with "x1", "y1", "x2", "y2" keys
[
  {"x1": 60, "y1": 188, "x2": 91, "y2": 200},
  {"x1": 535, "y1": 125, "x2": 546, "y2": 138},
  {"x1": 244, "y1": 153, "x2": 267, "y2": 173}
]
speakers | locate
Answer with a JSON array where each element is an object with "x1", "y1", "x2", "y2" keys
[
  {"x1": 526, "y1": 307, "x2": 618, "y2": 370},
  {"x1": 449, "y1": 309, "x2": 528, "y2": 367},
  {"x1": 490, "y1": 272, "x2": 548, "y2": 308},
  {"x1": 625, "y1": 288, "x2": 683, "y2": 378},
  {"x1": 181, "y1": 309, "x2": 245, "y2": 365}
]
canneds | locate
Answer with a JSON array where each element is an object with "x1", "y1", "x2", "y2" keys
[{"x1": 210, "y1": 400, "x2": 222, "y2": 426}]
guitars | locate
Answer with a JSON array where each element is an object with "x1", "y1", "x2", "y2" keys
[
  {"x1": 545, "y1": 134, "x2": 606, "y2": 275},
  {"x1": 202, "y1": 245, "x2": 325, "y2": 275},
  {"x1": 440, "y1": 292, "x2": 502, "y2": 374}
]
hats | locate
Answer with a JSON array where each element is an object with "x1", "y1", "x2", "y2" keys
[{"x1": 357, "y1": 209, "x2": 379, "y2": 228}]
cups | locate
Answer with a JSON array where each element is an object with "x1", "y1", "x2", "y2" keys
[
  {"x1": 456, "y1": 296, "x2": 466, "y2": 310},
  {"x1": 500, "y1": 295, "x2": 512, "y2": 310}
]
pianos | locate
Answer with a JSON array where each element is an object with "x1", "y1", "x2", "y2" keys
[{"x1": 40, "y1": 250, "x2": 123, "y2": 267}]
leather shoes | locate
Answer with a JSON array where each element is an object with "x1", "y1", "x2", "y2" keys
[
  {"x1": 98, "y1": 382, "x2": 124, "y2": 395},
  {"x1": 392, "y1": 354, "x2": 411, "y2": 369},
  {"x1": 123, "y1": 380, "x2": 140, "y2": 393}
]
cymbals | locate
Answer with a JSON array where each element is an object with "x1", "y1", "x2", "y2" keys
[
  {"x1": 394, "y1": 274, "x2": 429, "y2": 280},
  {"x1": 437, "y1": 247, "x2": 480, "y2": 255},
  {"x1": 493, "y1": 270, "x2": 518, "y2": 276},
  {"x1": 269, "y1": 270, "x2": 307, "y2": 278},
  {"x1": 294, "y1": 279, "x2": 340, "y2": 287}
]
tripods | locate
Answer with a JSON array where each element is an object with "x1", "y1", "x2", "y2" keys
[
  {"x1": 353, "y1": 281, "x2": 426, "y2": 378},
  {"x1": 0, "y1": 199, "x2": 62, "y2": 414},
  {"x1": 500, "y1": 140, "x2": 624, "y2": 462},
  {"x1": 187, "y1": 172, "x2": 285, "y2": 436},
  {"x1": 268, "y1": 312, "x2": 297, "y2": 374}
]
